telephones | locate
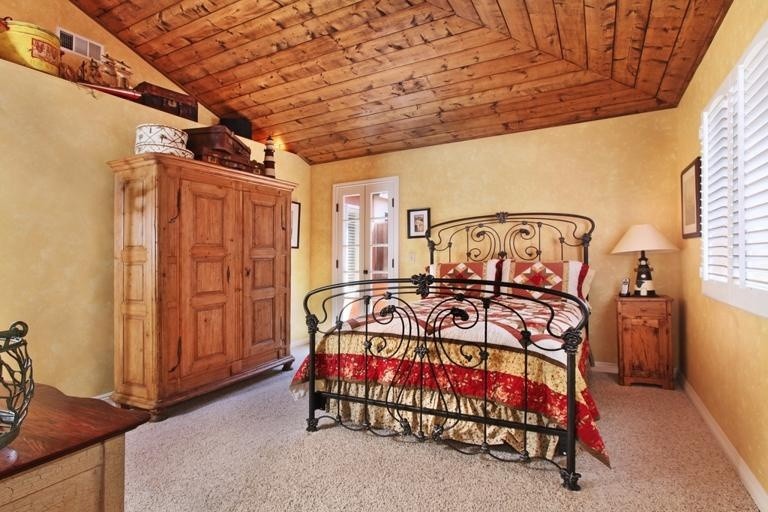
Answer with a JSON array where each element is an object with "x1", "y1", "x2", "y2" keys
[{"x1": 620, "y1": 277, "x2": 630, "y2": 296}]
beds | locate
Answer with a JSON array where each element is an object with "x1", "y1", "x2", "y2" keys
[{"x1": 288, "y1": 212, "x2": 612, "y2": 491}]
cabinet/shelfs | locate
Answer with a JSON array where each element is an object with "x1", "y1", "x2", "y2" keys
[{"x1": 107, "y1": 152, "x2": 300, "y2": 421}]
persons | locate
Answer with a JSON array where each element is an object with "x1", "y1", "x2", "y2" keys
[{"x1": 414, "y1": 218, "x2": 424, "y2": 232}]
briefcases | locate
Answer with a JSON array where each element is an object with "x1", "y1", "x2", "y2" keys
[{"x1": 182, "y1": 126, "x2": 265, "y2": 177}]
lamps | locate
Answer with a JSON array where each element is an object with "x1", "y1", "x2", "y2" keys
[{"x1": 610, "y1": 224, "x2": 681, "y2": 298}]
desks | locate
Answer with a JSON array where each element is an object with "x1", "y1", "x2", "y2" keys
[{"x1": 0, "y1": 381, "x2": 151, "y2": 511}]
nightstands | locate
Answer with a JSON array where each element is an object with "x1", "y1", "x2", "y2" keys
[{"x1": 614, "y1": 295, "x2": 674, "y2": 390}]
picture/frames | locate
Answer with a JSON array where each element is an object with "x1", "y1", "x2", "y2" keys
[
  {"x1": 407, "y1": 208, "x2": 430, "y2": 238},
  {"x1": 291, "y1": 201, "x2": 301, "y2": 248},
  {"x1": 681, "y1": 156, "x2": 701, "y2": 239}
]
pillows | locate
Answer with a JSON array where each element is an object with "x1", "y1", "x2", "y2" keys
[{"x1": 429, "y1": 259, "x2": 589, "y2": 305}]
